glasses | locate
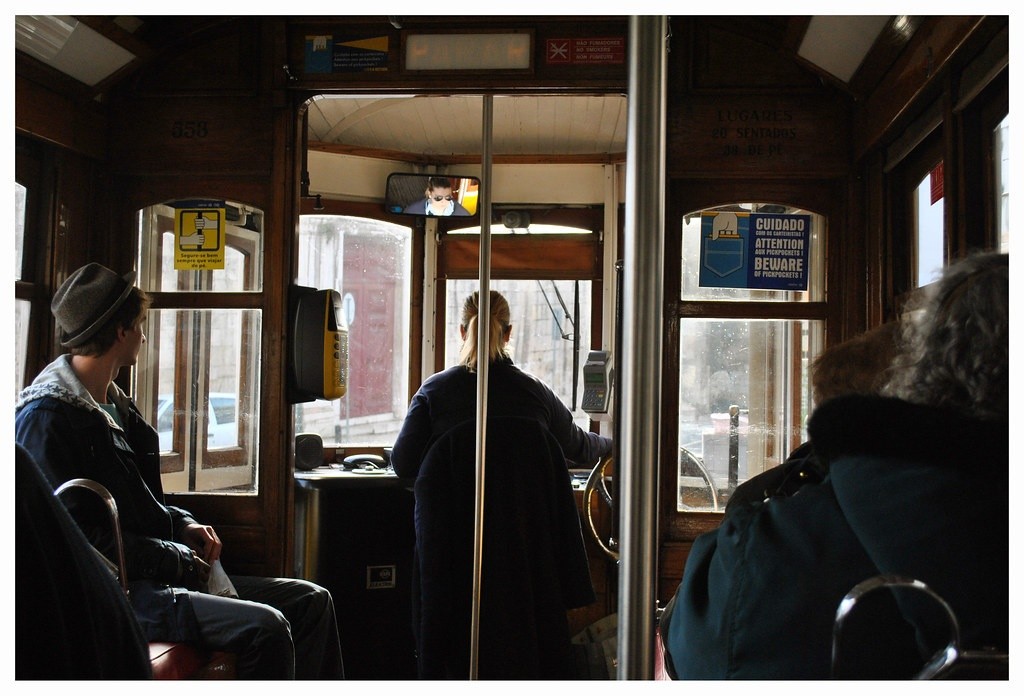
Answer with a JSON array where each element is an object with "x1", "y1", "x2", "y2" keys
[{"x1": 432, "y1": 194, "x2": 454, "y2": 201}]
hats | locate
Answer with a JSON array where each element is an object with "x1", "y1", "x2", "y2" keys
[{"x1": 51, "y1": 262, "x2": 138, "y2": 347}]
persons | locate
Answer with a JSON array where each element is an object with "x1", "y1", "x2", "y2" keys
[
  {"x1": 15, "y1": 263, "x2": 345, "y2": 681},
  {"x1": 660, "y1": 254, "x2": 1009, "y2": 681},
  {"x1": 403, "y1": 177, "x2": 471, "y2": 216},
  {"x1": 392, "y1": 292, "x2": 613, "y2": 481}
]
